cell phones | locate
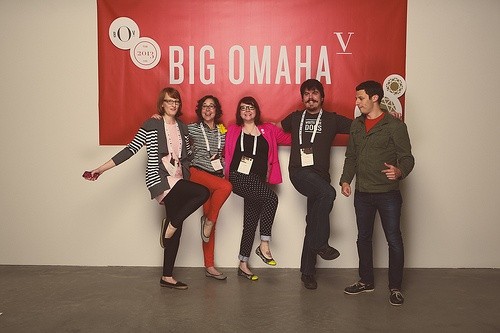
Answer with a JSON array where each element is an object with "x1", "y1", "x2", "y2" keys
[{"x1": 83, "y1": 171, "x2": 99, "y2": 180}]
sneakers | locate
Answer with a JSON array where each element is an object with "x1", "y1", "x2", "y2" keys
[
  {"x1": 389, "y1": 289, "x2": 404, "y2": 305},
  {"x1": 344, "y1": 280, "x2": 375, "y2": 295}
]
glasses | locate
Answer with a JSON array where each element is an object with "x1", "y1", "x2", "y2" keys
[
  {"x1": 202, "y1": 105, "x2": 215, "y2": 110},
  {"x1": 240, "y1": 106, "x2": 255, "y2": 110},
  {"x1": 164, "y1": 100, "x2": 180, "y2": 104}
]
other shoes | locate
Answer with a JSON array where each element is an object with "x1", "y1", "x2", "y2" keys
[
  {"x1": 205, "y1": 269, "x2": 227, "y2": 280},
  {"x1": 300, "y1": 272, "x2": 317, "y2": 288},
  {"x1": 201, "y1": 216, "x2": 210, "y2": 243},
  {"x1": 314, "y1": 245, "x2": 340, "y2": 260},
  {"x1": 238, "y1": 267, "x2": 258, "y2": 281},
  {"x1": 255, "y1": 245, "x2": 276, "y2": 266},
  {"x1": 160, "y1": 218, "x2": 172, "y2": 248},
  {"x1": 159, "y1": 276, "x2": 188, "y2": 290}
]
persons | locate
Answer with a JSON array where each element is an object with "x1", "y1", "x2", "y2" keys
[
  {"x1": 339, "y1": 81, "x2": 415, "y2": 305},
  {"x1": 265, "y1": 79, "x2": 403, "y2": 290},
  {"x1": 86, "y1": 86, "x2": 210, "y2": 290},
  {"x1": 151, "y1": 94, "x2": 233, "y2": 280},
  {"x1": 223, "y1": 96, "x2": 291, "y2": 280}
]
